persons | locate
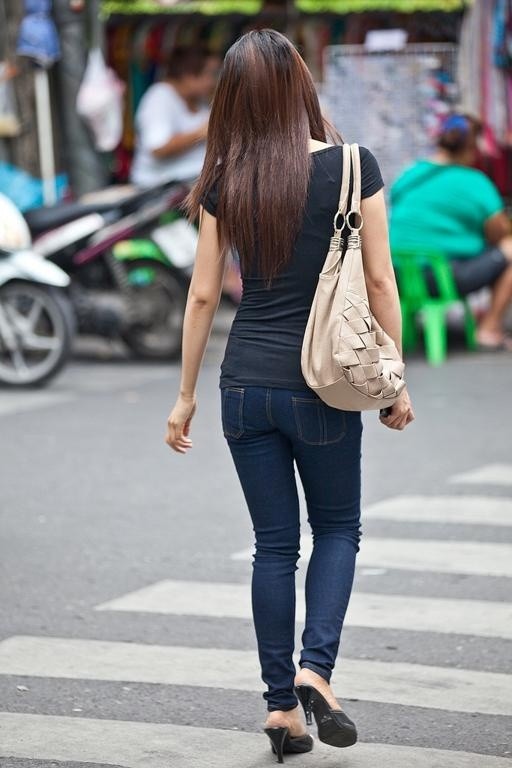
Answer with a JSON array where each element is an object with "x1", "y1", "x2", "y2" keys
[
  {"x1": 387, "y1": 113, "x2": 512, "y2": 351},
  {"x1": 167, "y1": 28, "x2": 416, "y2": 763},
  {"x1": 130, "y1": 46, "x2": 224, "y2": 183}
]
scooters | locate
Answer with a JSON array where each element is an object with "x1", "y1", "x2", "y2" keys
[
  {"x1": 1, "y1": 169, "x2": 105, "y2": 396},
  {"x1": 16, "y1": 173, "x2": 201, "y2": 365}
]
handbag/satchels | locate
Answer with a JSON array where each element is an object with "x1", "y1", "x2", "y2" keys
[{"x1": 296, "y1": 140, "x2": 408, "y2": 413}]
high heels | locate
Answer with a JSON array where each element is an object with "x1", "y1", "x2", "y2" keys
[
  {"x1": 262, "y1": 724, "x2": 315, "y2": 764},
  {"x1": 293, "y1": 682, "x2": 358, "y2": 747}
]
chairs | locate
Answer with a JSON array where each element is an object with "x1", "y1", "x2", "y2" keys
[{"x1": 388, "y1": 239, "x2": 478, "y2": 366}]
